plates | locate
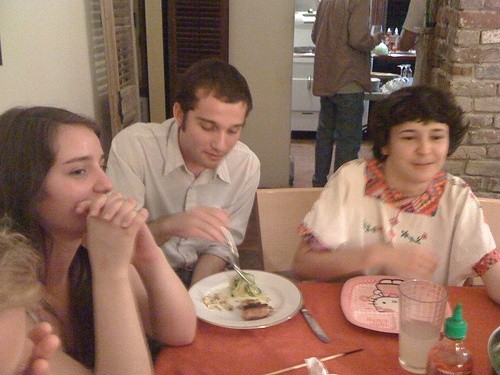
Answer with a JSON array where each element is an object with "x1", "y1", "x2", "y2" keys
[
  {"x1": 339, "y1": 275, "x2": 453, "y2": 334},
  {"x1": 189, "y1": 268, "x2": 302, "y2": 331},
  {"x1": 487, "y1": 326, "x2": 500, "y2": 375}
]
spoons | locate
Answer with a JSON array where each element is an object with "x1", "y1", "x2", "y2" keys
[{"x1": 221, "y1": 226, "x2": 253, "y2": 285}]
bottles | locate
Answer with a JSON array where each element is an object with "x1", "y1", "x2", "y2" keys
[
  {"x1": 392, "y1": 28, "x2": 400, "y2": 51},
  {"x1": 424, "y1": 303, "x2": 474, "y2": 375},
  {"x1": 403, "y1": 65, "x2": 413, "y2": 77},
  {"x1": 384, "y1": 28, "x2": 392, "y2": 51}
]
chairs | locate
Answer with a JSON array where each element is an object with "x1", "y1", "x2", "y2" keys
[
  {"x1": 470, "y1": 197, "x2": 500, "y2": 285},
  {"x1": 255, "y1": 187, "x2": 324, "y2": 273}
]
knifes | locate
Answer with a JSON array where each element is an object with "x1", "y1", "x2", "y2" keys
[{"x1": 301, "y1": 304, "x2": 329, "y2": 343}]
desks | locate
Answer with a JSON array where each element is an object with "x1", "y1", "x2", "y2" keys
[{"x1": 153, "y1": 282, "x2": 500, "y2": 375}]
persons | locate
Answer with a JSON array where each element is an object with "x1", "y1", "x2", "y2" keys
[
  {"x1": 291, "y1": 86, "x2": 500, "y2": 306},
  {"x1": 104, "y1": 57, "x2": 260, "y2": 290},
  {"x1": 397, "y1": 0, "x2": 426, "y2": 52},
  {"x1": 0, "y1": 106, "x2": 197, "y2": 375},
  {"x1": 312, "y1": 0, "x2": 385, "y2": 187}
]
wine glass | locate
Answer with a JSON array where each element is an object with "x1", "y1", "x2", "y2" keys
[{"x1": 397, "y1": 64, "x2": 407, "y2": 87}]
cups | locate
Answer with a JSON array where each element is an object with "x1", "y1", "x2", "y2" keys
[
  {"x1": 397, "y1": 278, "x2": 447, "y2": 374},
  {"x1": 371, "y1": 77, "x2": 381, "y2": 92}
]
